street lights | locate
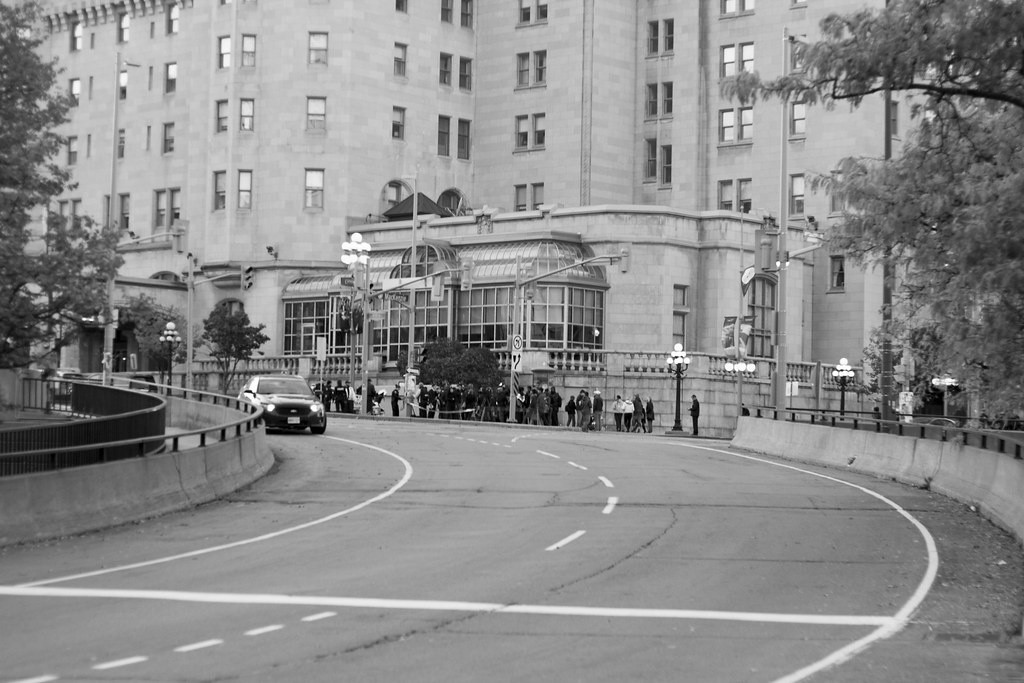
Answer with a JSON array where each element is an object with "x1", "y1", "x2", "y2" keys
[
  {"x1": 667, "y1": 343, "x2": 690, "y2": 432},
  {"x1": 341, "y1": 233, "x2": 371, "y2": 393},
  {"x1": 932, "y1": 373, "x2": 959, "y2": 415},
  {"x1": 726, "y1": 358, "x2": 756, "y2": 436},
  {"x1": 831, "y1": 358, "x2": 855, "y2": 421},
  {"x1": 159, "y1": 322, "x2": 181, "y2": 396}
]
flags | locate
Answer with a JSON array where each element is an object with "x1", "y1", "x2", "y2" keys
[
  {"x1": 738, "y1": 317, "x2": 753, "y2": 359},
  {"x1": 721, "y1": 317, "x2": 736, "y2": 360}
]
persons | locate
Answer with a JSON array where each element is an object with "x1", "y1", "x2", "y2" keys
[
  {"x1": 566, "y1": 389, "x2": 603, "y2": 433},
  {"x1": 742, "y1": 402, "x2": 750, "y2": 416},
  {"x1": 391, "y1": 384, "x2": 403, "y2": 416},
  {"x1": 612, "y1": 392, "x2": 655, "y2": 434},
  {"x1": 417, "y1": 382, "x2": 562, "y2": 427},
  {"x1": 978, "y1": 408, "x2": 1022, "y2": 431},
  {"x1": 687, "y1": 394, "x2": 700, "y2": 436},
  {"x1": 312, "y1": 378, "x2": 379, "y2": 415}
]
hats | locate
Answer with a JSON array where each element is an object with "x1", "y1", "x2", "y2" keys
[
  {"x1": 346, "y1": 381, "x2": 351, "y2": 383},
  {"x1": 594, "y1": 391, "x2": 601, "y2": 395}
]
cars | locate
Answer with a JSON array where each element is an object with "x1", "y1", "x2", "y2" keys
[
  {"x1": 47, "y1": 370, "x2": 84, "y2": 403},
  {"x1": 238, "y1": 374, "x2": 327, "y2": 435},
  {"x1": 908, "y1": 416, "x2": 958, "y2": 428}
]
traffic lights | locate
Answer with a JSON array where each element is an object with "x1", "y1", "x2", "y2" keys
[{"x1": 240, "y1": 264, "x2": 255, "y2": 293}]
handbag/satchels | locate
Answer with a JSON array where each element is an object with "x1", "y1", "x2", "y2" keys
[{"x1": 565, "y1": 406, "x2": 570, "y2": 411}]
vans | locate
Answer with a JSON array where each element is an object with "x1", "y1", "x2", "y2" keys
[{"x1": 87, "y1": 373, "x2": 158, "y2": 394}]
